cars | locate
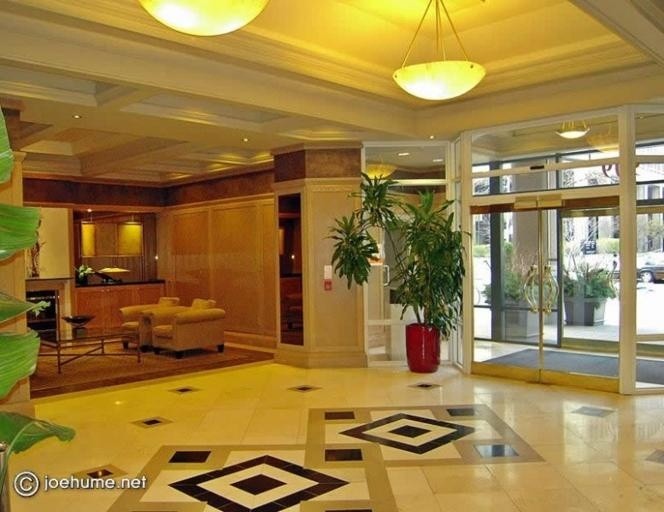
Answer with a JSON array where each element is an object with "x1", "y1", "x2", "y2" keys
[{"x1": 588, "y1": 252, "x2": 664, "y2": 283}]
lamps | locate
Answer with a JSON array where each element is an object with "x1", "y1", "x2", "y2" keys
[
  {"x1": 392, "y1": 1, "x2": 486, "y2": 102},
  {"x1": 365, "y1": 164, "x2": 396, "y2": 179},
  {"x1": 138, "y1": 0, "x2": 273, "y2": 37},
  {"x1": 554, "y1": 121, "x2": 589, "y2": 141}
]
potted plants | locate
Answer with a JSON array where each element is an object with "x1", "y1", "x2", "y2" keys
[
  {"x1": 479, "y1": 243, "x2": 556, "y2": 338},
  {"x1": 330, "y1": 171, "x2": 468, "y2": 373},
  {"x1": 562, "y1": 265, "x2": 617, "y2": 326}
]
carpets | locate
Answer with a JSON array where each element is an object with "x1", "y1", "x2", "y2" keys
[
  {"x1": 480, "y1": 347, "x2": 664, "y2": 387},
  {"x1": 30, "y1": 342, "x2": 274, "y2": 398}
]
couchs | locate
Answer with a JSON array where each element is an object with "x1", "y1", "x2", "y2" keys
[
  {"x1": 117, "y1": 296, "x2": 180, "y2": 352},
  {"x1": 146, "y1": 297, "x2": 226, "y2": 360}
]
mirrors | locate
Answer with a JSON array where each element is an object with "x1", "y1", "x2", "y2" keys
[{"x1": 277, "y1": 192, "x2": 304, "y2": 346}]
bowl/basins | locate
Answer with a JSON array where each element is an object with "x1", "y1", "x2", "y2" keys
[{"x1": 60, "y1": 314, "x2": 96, "y2": 328}]
